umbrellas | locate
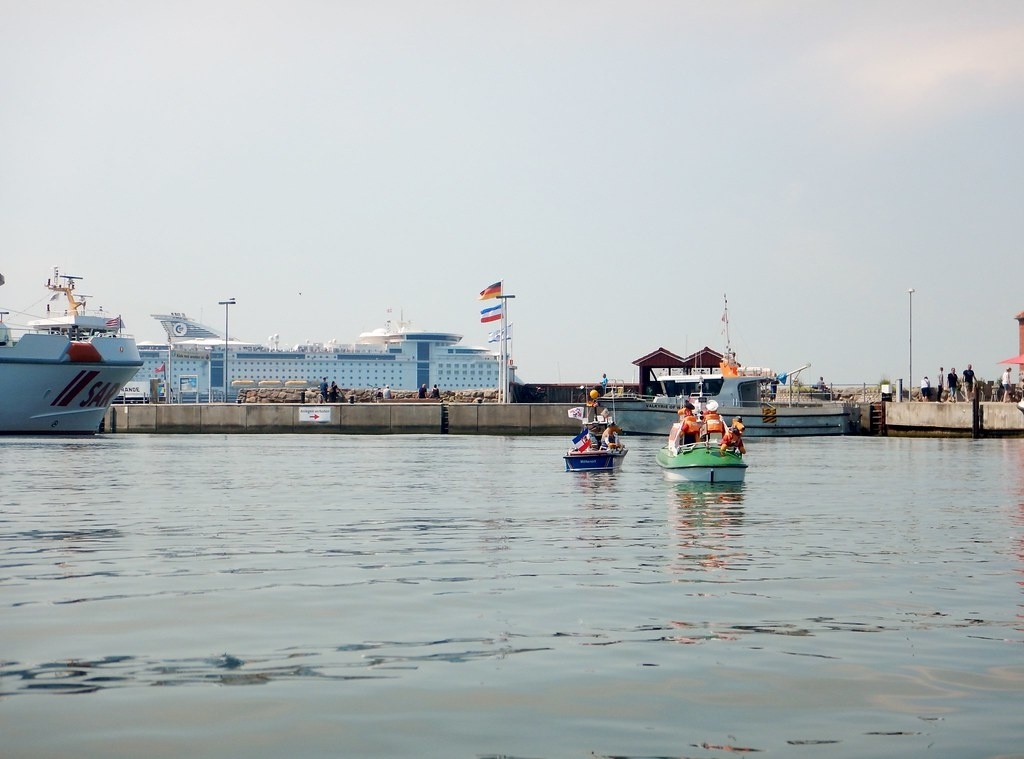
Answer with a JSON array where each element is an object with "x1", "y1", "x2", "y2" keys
[{"x1": 998, "y1": 354, "x2": 1024, "y2": 364}]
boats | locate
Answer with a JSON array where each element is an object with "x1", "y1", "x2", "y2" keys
[
  {"x1": 594, "y1": 291, "x2": 862, "y2": 437},
  {"x1": 656, "y1": 375, "x2": 749, "y2": 483},
  {"x1": 563, "y1": 388, "x2": 628, "y2": 472},
  {"x1": 0, "y1": 266, "x2": 145, "y2": 435}
]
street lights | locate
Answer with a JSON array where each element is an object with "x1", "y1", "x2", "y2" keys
[
  {"x1": 495, "y1": 295, "x2": 515, "y2": 402},
  {"x1": 219, "y1": 301, "x2": 236, "y2": 403},
  {"x1": 906, "y1": 288, "x2": 916, "y2": 402}
]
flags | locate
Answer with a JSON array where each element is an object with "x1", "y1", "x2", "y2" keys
[
  {"x1": 155, "y1": 364, "x2": 164, "y2": 372},
  {"x1": 567, "y1": 406, "x2": 584, "y2": 419},
  {"x1": 489, "y1": 325, "x2": 511, "y2": 343},
  {"x1": 106, "y1": 317, "x2": 120, "y2": 328},
  {"x1": 480, "y1": 303, "x2": 503, "y2": 322},
  {"x1": 571, "y1": 429, "x2": 591, "y2": 453},
  {"x1": 480, "y1": 282, "x2": 501, "y2": 300}
]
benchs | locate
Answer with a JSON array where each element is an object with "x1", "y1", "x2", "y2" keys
[{"x1": 372, "y1": 399, "x2": 443, "y2": 403}]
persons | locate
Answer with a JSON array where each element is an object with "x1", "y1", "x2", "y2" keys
[
  {"x1": 937, "y1": 364, "x2": 978, "y2": 403},
  {"x1": 817, "y1": 377, "x2": 826, "y2": 392},
  {"x1": 431, "y1": 384, "x2": 440, "y2": 398},
  {"x1": 320, "y1": 378, "x2": 338, "y2": 403},
  {"x1": 377, "y1": 388, "x2": 383, "y2": 397},
  {"x1": 1002, "y1": 367, "x2": 1011, "y2": 403},
  {"x1": 383, "y1": 385, "x2": 391, "y2": 398},
  {"x1": 418, "y1": 384, "x2": 428, "y2": 399},
  {"x1": 600, "y1": 375, "x2": 607, "y2": 393},
  {"x1": 921, "y1": 377, "x2": 930, "y2": 401}
]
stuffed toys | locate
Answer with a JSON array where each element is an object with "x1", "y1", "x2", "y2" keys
[
  {"x1": 720, "y1": 416, "x2": 745, "y2": 455},
  {"x1": 600, "y1": 421, "x2": 622, "y2": 450},
  {"x1": 676, "y1": 400, "x2": 724, "y2": 443}
]
